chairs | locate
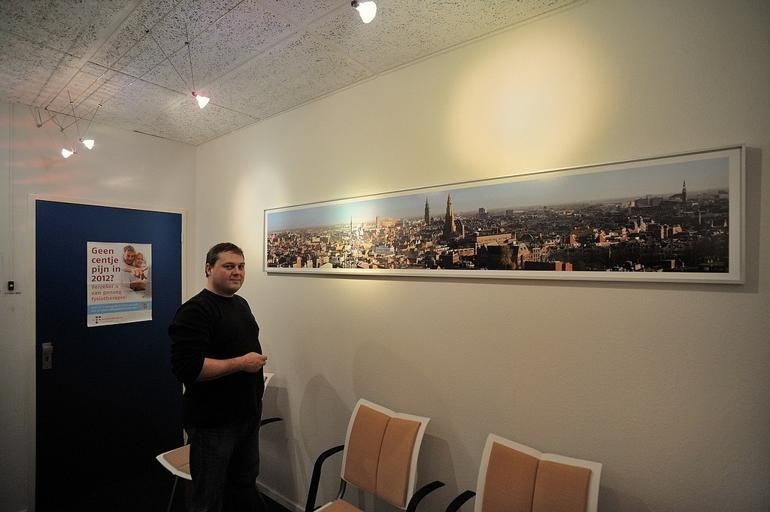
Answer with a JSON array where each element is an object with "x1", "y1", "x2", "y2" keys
[{"x1": 156, "y1": 365, "x2": 284, "y2": 511}]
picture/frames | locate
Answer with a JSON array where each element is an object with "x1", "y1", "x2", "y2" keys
[{"x1": 262, "y1": 143, "x2": 748, "y2": 293}]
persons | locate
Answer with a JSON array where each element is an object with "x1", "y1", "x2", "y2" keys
[
  {"x1": 131, "y1": 252, "x2": 145, "y2": 268},
  {"x1": 167, "y1": 242, "x2": 270, "y2": 510},
  {"x1": 120, "y1": 245, "x2": 150, "y2": 283}
]
